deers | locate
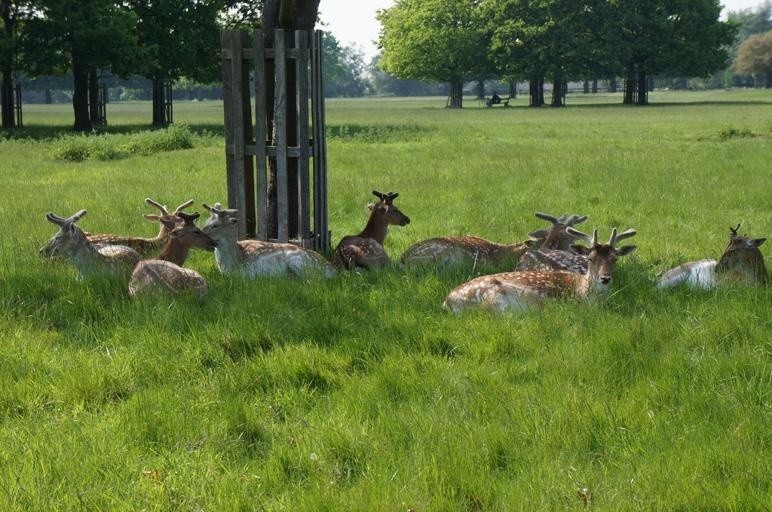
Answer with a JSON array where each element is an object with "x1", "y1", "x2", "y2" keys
[
  {"x1": 200, "y1": 202, "x2": 339, "y2": 283},
  {"x1": 39, "y1": 207, "x2": 143, "y2": 285},
  {"x1": 514, "y1": 227, "x2": 593, "y2": 276},
  {"x1": 332, "y1": 189, "x2": 411, "y2": 272},
  {"x1": 658, "y1": 223, "x2": 769, "y2": 293},
  {"x1": 83, "y1": 198, "x2": 196, "y2": 259},
  {"x1": 128, "y1": 213, "x2": 219, "y2": 301},
  {"x1": 442, "y1": 227, "x2": 635, "y2": 320},
  {"x1": 400, "y1": 211, "x2": 589, "y2": 271}
]
